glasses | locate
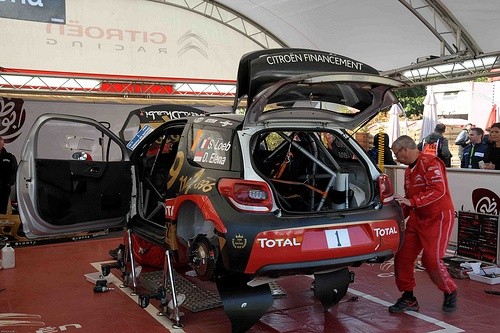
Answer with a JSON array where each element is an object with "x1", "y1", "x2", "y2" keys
[{"x1": 394, "y1": 146, "x2": 403, "y2": 156}]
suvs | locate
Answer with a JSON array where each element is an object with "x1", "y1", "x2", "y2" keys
[{"x1": 15, "y1": 48, "x2": 410, "y2": 331}]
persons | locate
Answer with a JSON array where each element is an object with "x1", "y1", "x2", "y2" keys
[
  {"x1": 366, "y1": 132, "x2": 397, "y2": 166},
  {"x1": 0, "y1": 136, "x2": 18, "y2": 214},
  {"x1": 418, "y1": 123, "x2": 453, "y2": 167},
  {"x1": 482, "y1": 122, "x2": 500, "y2": 169},
  {"x1": 388, "y1": 135, "x2": 458, "y2": 313},
  {"x1": 460, "y1": 127, "x2": 488, "y2": 169},
  {"x1": 455, "y1": 123, "x2": 473, "y2": 167}
]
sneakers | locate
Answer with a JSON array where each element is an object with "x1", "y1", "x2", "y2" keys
[
  {"x1": 389, "y1": 296, "x2": 419, "y2": 313},
  {"x1": 442, "y1": 289, "x2": 457, "y2": 313}
]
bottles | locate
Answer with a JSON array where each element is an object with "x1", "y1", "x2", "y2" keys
[{"x1": 0, "y1": 243, "x2": 15, "y2": 269}]
